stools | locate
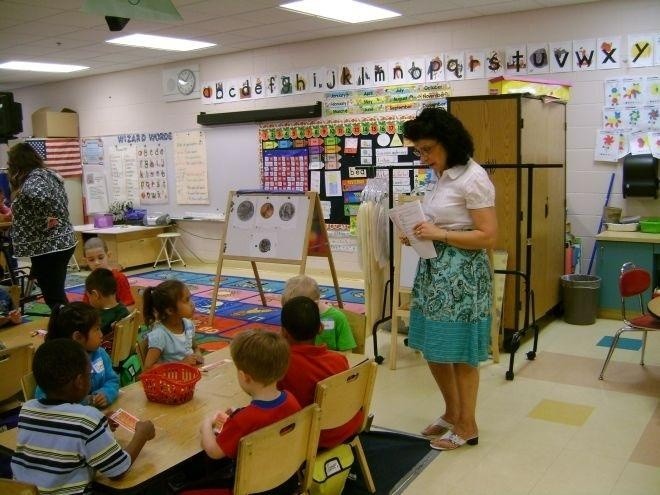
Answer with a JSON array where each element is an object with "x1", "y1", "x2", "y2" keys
[
  {"x1": 153, "y1": 231, "x2": 188, "y2": 271},
  {"x1": 66, "y1": 252, "x2": 81, "y2": 273}
]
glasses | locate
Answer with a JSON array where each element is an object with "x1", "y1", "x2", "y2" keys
[{"x1": 413, "y1": 143, "x2": 440, "y2": 157}]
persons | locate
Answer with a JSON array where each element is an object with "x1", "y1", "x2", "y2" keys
[
  {"x1": 396, "y1": 106, "x2": 499, "y2": 451},
  {"x1": 7, "y1": 143, "x2": 77, "y2": 310},
  {"x1": 276, "y1": 297, "x2": 364, "y2": 453},
  {"x1": 0, "y1": 265, "x2": 22, "y2": 328},
  {"x1": 87, "y1": 268, "x2": 129, "y2": 355},
  {"x1": 175, "y1": 330, "x2": 305, "y2": 495},
  {"x1": 1, "y1": 187, "x2": 12, "y2": 224},
  {"x1": 142, "y1": 281, "x2": 204, "y2": 371},
  {"x1": 12, "y1": 337, "x2": 154, "y2": 493},
  {"x1": 81, "y1": 238, "x2": 135, "y2": 312},
  {"x1": 282, "y1": 276, "x2": 357, "y2": 353},
  {"x1": 34, "y1": 300, "x2": 120, "y2": 410}
]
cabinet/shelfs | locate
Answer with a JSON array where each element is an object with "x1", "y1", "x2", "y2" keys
[
  {"x1": 7, "y1": 137, "x2": 86, "y2": 226},
  {"x1": 444, "y1": 93, "x2": 568, "y2": 352},
  {"x1": 593, "y1": 229, "x2": 659, "y2": 324}
]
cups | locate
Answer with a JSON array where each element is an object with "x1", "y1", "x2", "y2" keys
[{"x1": 604, "y1": 207, "x2": 622, "y2": 228}]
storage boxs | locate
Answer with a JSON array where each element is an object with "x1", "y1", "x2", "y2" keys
[
  {"x1": 483, "y1": 73, "x2": 574, "y2": 104},
  {"x1": 30, "y1": 103, "x2": 81, "y2": 138}
]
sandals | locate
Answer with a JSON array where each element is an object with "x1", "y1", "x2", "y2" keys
[
  {"x1": 422, "y1": 417, "x2": 455, "y2": 435},
  {"x1": 429, "y1": 428, "x2": 479, "y2": 450}
]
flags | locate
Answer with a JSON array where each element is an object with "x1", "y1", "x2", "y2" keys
[{"x1": 26, "y1": 139, "x2": 83, "y2": 177}]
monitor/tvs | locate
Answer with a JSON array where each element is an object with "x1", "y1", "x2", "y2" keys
[{"x1": 0, "y1": 91, "x2": 23, "y2": 141}]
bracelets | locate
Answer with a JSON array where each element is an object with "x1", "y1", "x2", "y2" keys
[{"x1": 444, "y1": 231, "x2": 448, "y2": 245}]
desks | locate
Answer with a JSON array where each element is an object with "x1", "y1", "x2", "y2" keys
[
  {"x1": 0, "y1": 339, "x2": 368, "y2": 490},
  {"x1": 68, "y1": 220, "x2": 179, "y2": 271},
  {"x1": 647, "y1": 295, "x2": 660, "y2": 321}
]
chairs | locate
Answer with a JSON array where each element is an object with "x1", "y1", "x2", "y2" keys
[
  {"x1": 232, "y1": 402, "x2": 323, "y2": 495},
  {"x1": 1, "y1": 265, "x2": 150, "y2": 435},
  {"x1": 333, "y1": 306, "x2": 367, "y2": 356},
  {"x1": 293, "y1": 357, "x2": 380, "y2": 493},
  {"x1": 598, "y1": 260, "x2": 660, "y2": 384},
  {"x1": 1, "y1": 478, "x2": 38, "y2": 494}
]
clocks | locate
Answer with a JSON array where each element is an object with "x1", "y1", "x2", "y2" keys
[{"x1": 174, "y1": 66, "x2": 197, "y2": 95}]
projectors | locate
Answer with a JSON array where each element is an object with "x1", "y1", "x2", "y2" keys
[{"x1": 147, "y1": 211, "x2": 171, "y2": 226}]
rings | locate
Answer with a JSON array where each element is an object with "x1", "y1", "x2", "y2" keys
[{"x1": 418, "y1": 231, "x2": 420, "y2": 233}]
